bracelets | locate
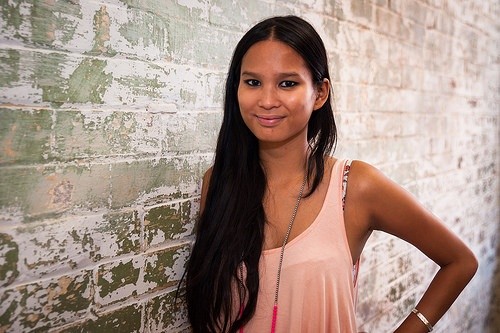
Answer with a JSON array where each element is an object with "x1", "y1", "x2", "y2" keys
[{"x1": 411, "y1": 308, "x2": 434, "y2": 333}]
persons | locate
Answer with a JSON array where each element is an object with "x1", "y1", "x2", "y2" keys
[{"x1": 183, "y1": 15, "x2": 478, "y2": 333}]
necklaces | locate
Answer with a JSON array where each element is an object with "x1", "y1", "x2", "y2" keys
[{"x1": 237, "y1": 169, "x2": 309, "y2": 333}]
